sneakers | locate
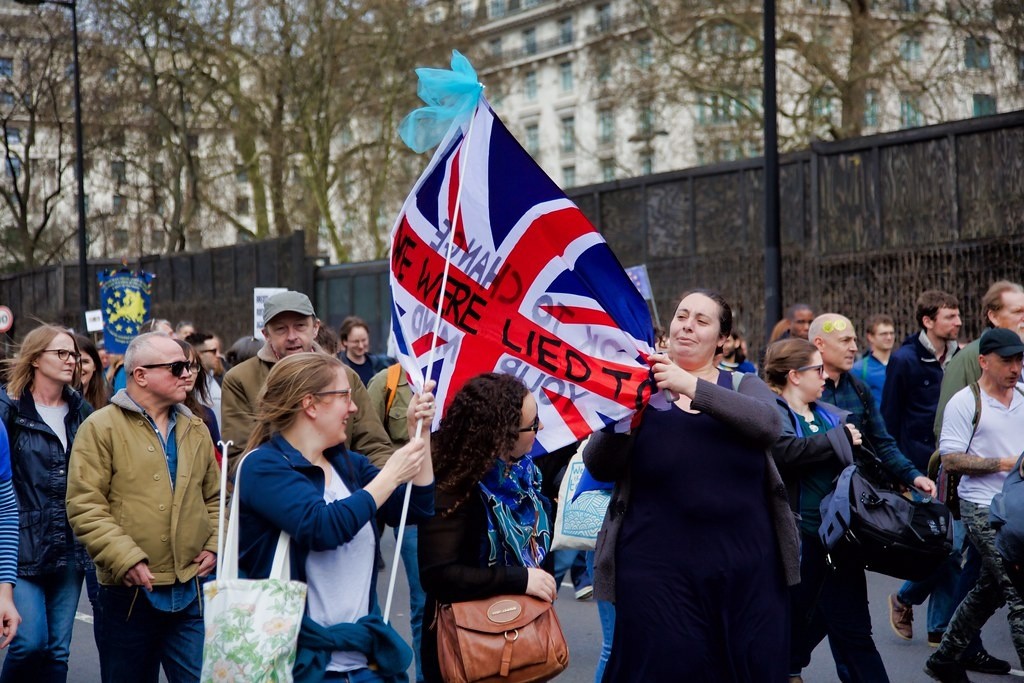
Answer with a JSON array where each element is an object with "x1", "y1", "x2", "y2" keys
[
  {"x1": 922, "y1": 652, "x2": 974, "y2": 683},
  {"x1": 962, "y1": 649, "x2": 1011, "y2": 675}
]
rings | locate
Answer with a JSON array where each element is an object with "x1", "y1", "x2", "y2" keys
[{"x1": 429, "y1": 402, "x2": 433, "y2": 408}]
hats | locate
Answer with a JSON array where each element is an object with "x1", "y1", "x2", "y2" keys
[
  {"x1": 262, "y1": 291, "x2": 315, "y2": 327},
  {"x1": 979, "y1": 328, "x2": 1024, "y2": 357}
]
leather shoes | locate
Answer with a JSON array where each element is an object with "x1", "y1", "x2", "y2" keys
[
  {"x1": 926, "y1": 632, "x2": 944, "y2": 647},
  {"x1": 888, "y1": 590, "x2": 915, "y2": 640}
]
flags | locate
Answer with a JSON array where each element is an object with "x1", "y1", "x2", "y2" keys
[{"x1": 390, "y1": 94, "x2": 654, "y2": 458}]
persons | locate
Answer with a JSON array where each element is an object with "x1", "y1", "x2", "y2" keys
[
  {"x1": 758, "y1": 301, "x2": 936, "y2": 683},
  {"x1": 878, "y1": 280, "x2": 1024, "y2": 683},
  {"x1": 221, "y1": 290, "x2": 412, "y2": 683},
  {"x1": 367, "y1": 362, "x2": 556, "y2": 683},
  {"x1": 0, "y1": 318, "x2": 227, "y2": 683},
  {"x1": 531, "y1": 288, "x2": 782, "y2": 683},
  {"x1": 850, "y1": 312, "x2": 896, "y2": 409}
]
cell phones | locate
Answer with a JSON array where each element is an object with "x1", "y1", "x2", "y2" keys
[{"x1": 659, "y1": 351, "x2": 680, "y2": 403}]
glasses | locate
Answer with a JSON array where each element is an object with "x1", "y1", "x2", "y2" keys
[
  {"x1": 516, "y1": 417, "x2": 540, "y2": 435},
  {"x1": 190, "y1": 364, "x2": 201, "y2": 373},
  {"x1": 797, "y1": 364, "x2": 825, "y2": 377},
  {"x1": 41, "y1": 348, "x2": 81, "y2": 363},
  {"x1": 200, "y1": 348, "x2": 218, "y2": 355},
  {"x1": 129, "y1": 360, "x2": 191, "y2": 377},
  {"x1": 312, "y1": 388, "x2": 352, "y2": 403}
]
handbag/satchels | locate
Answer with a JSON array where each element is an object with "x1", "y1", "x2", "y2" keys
[
  {"x1": 428, "y1": 587, "x2": 569, "y2": 683},
  {"x1": 200, "y1": 577, "x2": 308, "y2": 683},
  {"x1": 550, "y1": 434, "x2": 614, "y2": 551},
  {"x1": 820, "y1": 430, "x2": 954, "y2": 582},
  {"x1": 987, "y1": 452, "x2": 1024, "y2": 564},
  {"x1": 937, "y1": 463, "x2": 961, "y2": 508}
]
